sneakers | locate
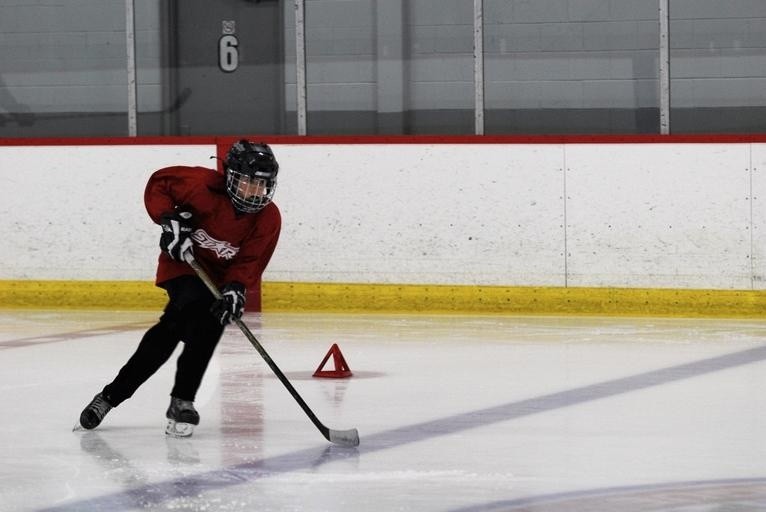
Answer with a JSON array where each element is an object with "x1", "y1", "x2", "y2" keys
[
  {"x1": 80, "y1": 392, "x2": 114, "y2": 430},
  {"x1": 165, "y1": 395, "x2": 200, "y2": 425}
]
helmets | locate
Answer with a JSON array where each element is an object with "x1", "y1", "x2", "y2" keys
[{"x1": 223, "y1": 138, "x2": 279, "y2": 216}]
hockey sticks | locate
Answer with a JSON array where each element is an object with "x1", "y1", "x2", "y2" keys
[{"x1": 184, "y1": 251, "x2": 359, "y2": 446}]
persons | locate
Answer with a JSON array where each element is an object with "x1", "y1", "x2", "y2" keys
[{"x1": 80, "y1": 139, "x2": 282, "y2": 430}]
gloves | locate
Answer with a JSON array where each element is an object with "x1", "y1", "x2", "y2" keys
[
  {"x1": 158, "y1": 212, "x2": 196, "y2": 263},
  {"x1": 211, "y1": 281, "x2": 247, "y2": 326}
]
